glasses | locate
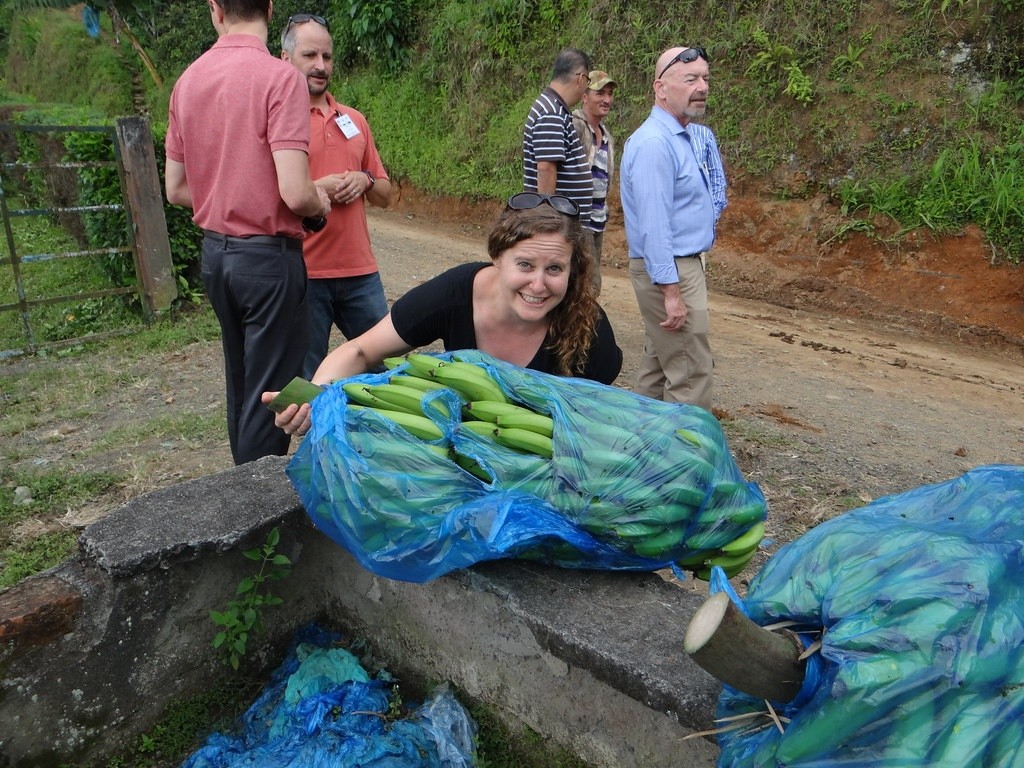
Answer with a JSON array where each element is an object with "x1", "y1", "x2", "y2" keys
[
  {"x1": 504, "y1": 192, "x2": 579, "y2": 222},
  {"x1": 283, "y1": 14, "x2": 330, "y2": 46},
  {"x1": 576, "y1": 74, "x2": 591, "y2": 86},
  {"x1": 659, "y1": 48, "x2": 708, "y2": 79}
]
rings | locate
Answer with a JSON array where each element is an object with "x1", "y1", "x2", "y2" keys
[{"x1": 351, "y1": 190, "x2": 356, "y2": 194}]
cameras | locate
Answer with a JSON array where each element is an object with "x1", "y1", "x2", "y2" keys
[{"x1": 302, "y1": 215, "x2": 327, "y2": 233}]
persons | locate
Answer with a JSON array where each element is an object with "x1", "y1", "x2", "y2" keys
[
  {"x1": 570, "y1": 69, "x2": 617, "y2": 263},
  {"x1": 163, "y1": 0, "x2": 332, "y2": 467},
  {"x1": 260, "y1": 192, "x2": 623, "y2": 438},
  {"x1": 618, "y1": 46, "x2": 727, "y2": 418},
  {"x1": 522, "y1": 48, "x2": 602, "y2": 302},
  {"x1": 278, "y1": 14, "x2": 393, "y2": 384}
]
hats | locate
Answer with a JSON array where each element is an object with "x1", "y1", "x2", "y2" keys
[{"x1": 587, "y1": 71, "x2": 618, "y2": 90}]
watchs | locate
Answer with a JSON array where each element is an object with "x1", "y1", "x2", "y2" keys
[{"x1": 362, "y1": 170, "x2": 376, "y2": 194}]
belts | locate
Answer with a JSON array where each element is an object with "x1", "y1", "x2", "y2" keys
[{"x1": 204, "y1": 229, "x2": 302, "y2": 249}]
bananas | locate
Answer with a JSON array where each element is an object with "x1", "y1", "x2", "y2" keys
[
  {"x1": 291, "y1": 352, "x2": 764, "y2": 588},
  {"x1": 739, "y1": 465, "x2": 1024, "y2": 768}
]
